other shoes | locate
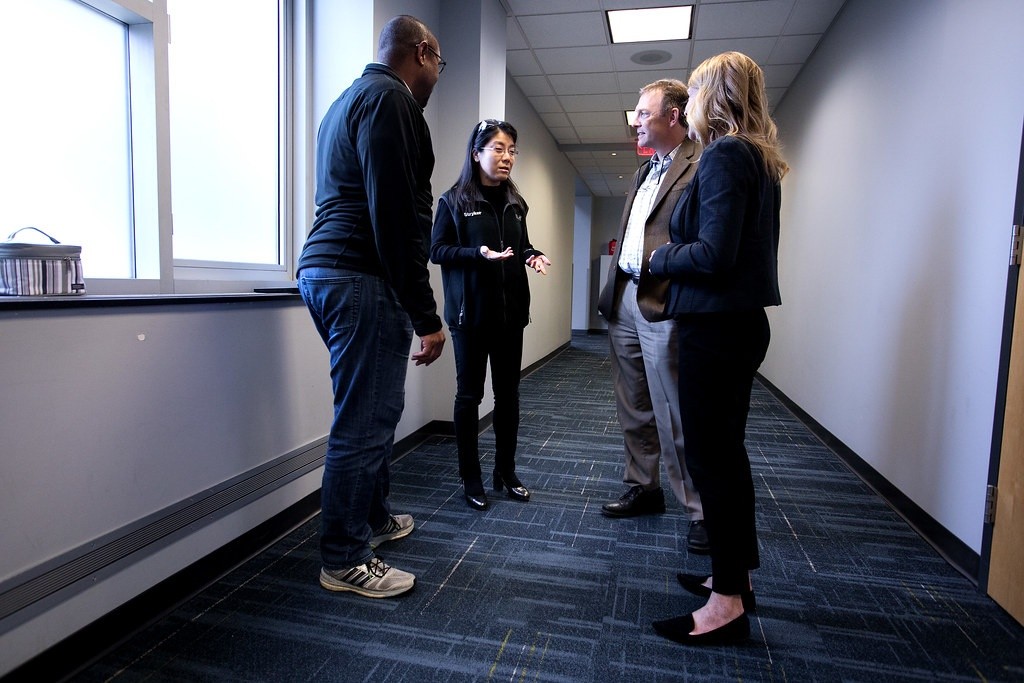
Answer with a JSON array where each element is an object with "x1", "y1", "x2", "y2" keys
[
  {"x1": 651, "y1": 610, "x2": 750, "y2": 646},
  {"x1": 676, "y1": 572, "x2": 757, "y2": 605}
]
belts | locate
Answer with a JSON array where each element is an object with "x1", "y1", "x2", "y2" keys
[{"x1": 623, "y1": 272, "x2": 640, "y2": 285}]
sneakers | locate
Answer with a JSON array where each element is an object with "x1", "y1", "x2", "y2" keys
[
  {"x1": 319, "y1": 557, "x2": 416, "y2": 599},
  {"x1": 369, "y1": 514, "x2": 414, "y2": 550}
]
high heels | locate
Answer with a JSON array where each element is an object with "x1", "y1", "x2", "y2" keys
[
  {"x1": 461, "y1": 479, "x2": 488, "y2": 511},
  {"x1": 492, "y1": 467, "x2": 531, "y2": 500}
]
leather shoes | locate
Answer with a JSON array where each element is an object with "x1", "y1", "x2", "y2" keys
[
  {"x1": 601, "y1": 486, "x2": 667, "y2": 517},
  {"x1": 686, "y1": 520, "x2": 711, "y2": 554}
]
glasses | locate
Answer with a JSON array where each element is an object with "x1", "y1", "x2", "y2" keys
[
  {"x1": 476, "y1": 119, "x2": 504, "y2": 137},
  {"x1": 415, "y1": 43, "x2": 447, "y2": 75},
  {"x1": 474, "y1": 145, "x2": 520, "y2": 158}
]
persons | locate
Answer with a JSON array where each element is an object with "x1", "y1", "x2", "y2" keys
[
  {"x1": 646, "y1": 51, "x2": 791, "y2": 646},
  {"x1": 431, "y1": 120, "x2": 553, "y2": 511},
  {"x1": 294, "y1": 15, "x2": 446, "y2": 600},
  {"x1": 596, "y1": 77, "x2": 715, "y2": 555}
]
